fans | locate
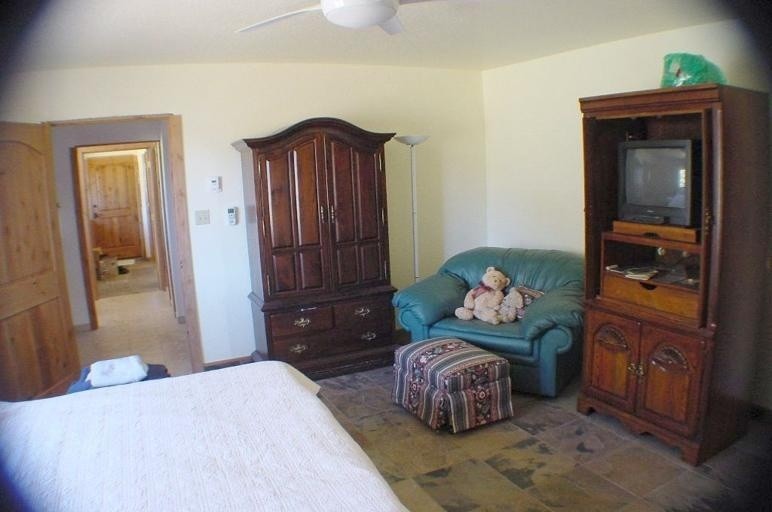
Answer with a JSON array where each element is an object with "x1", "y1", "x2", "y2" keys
[{"x1": 227, "y1": 0, "x2": 453, "y2": 58}]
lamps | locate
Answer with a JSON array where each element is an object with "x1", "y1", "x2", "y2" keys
[{"x1": 393, "y1": 134, "x2": 435, "y2": 281}]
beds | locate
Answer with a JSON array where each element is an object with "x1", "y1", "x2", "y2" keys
[{"x1": 0, "y1": 359, "x2": 410, "y2": 512}]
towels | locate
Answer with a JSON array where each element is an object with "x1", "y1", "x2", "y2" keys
[{"x1": 82, "y1": 356, "x2": 153, "y2": 386}]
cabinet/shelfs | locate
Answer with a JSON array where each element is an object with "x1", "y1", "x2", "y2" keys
[
  {"x1": 572, "y1": 83, "x2": 772, "y2": 467},
  {"x1": 230, "y1": 117, "x2": 397, "y2": 381}
]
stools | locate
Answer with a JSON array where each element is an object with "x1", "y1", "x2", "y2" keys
[{"x1": 391, "y1": 337, "x2": 514, "y2": 433}]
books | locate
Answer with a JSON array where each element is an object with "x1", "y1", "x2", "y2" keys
[{"x1": 624, "y1": 269, "x2": 660, "y2": 281}]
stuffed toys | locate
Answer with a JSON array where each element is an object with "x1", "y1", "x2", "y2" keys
[
  {"x1": 515, "y1": 284, "x2": 544, "y2": 318},
  {"x1": 494, "y1": 287, "x2": 526, "y2": 325},
  {"x1": 455, "y1": 267, "x2": 510, "y2": 326}
]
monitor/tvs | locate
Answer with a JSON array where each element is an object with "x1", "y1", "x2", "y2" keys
[{"x1": 617, "y1": 138, "x2": 701, "y2": 226}]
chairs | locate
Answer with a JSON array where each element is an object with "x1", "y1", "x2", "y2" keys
[{"x1": 401, "y1": 244, "x2": 583, "y2": 398}]
